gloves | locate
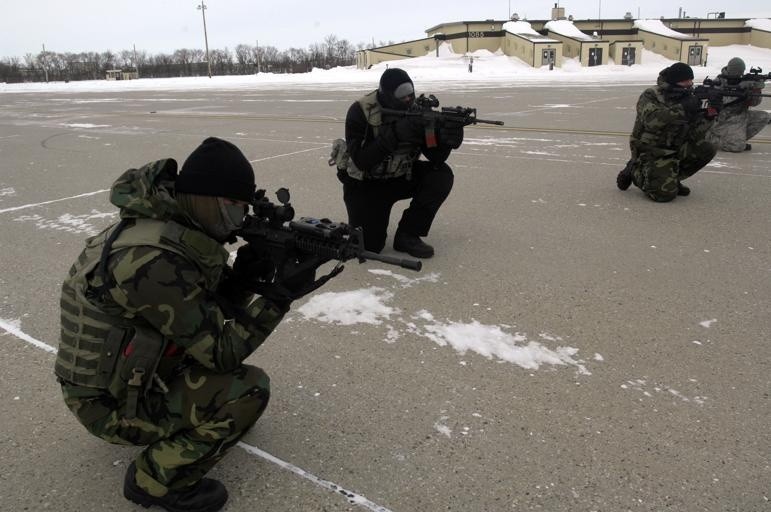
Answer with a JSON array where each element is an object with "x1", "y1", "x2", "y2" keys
[
  {"x1": 393, "y1": 117, "x2": 429, "y2": 143},
  {"x1": 682, "y1": 93, "x2": 701, "y2": 111},
  {"x1": 233, "y1": 245, "x2": 268, "y2": 284},
  {"x1": 267, "y1": 264, "x2": 314, "y2": 309},
  {"x1": 708, "y1": 93, "x2": 723, "y2": 110},
  {"x1": 440, "y1": 122, "x2": 463, "y2": 148}
]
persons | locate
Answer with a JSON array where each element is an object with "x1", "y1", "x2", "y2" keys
[
  {"x1": 334, "y1": 68, "x2": 464, "y2": 257},
  {"x1": 54, "y1": 136, "x2": 319, "y2": 511},
  {"x1": 714, "y1": 57, "x2": 771, "y2": 153},
  {"x1": 616, "y1": 63, "x2": 721, "y2": 202}
]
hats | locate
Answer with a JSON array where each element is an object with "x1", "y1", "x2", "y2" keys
[
  {"x1": 376, "y1": 69, "x2": 415, "y2": 110},
  {"x1": 728, "y1": 57, "x2": 745, "y2": 74},
  {"x1": 175, "y1": 136, "x2": 256, "y2": 201},
  {"x1": 665, "y1": 63, "x2": 694, "y2": 83}
]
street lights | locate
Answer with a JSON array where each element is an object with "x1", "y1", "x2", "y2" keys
[{"x1": 196, "y1": 0, "x2": 212, "y2": 78}]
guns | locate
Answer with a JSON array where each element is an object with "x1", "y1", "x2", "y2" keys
[
  {"x1": 717, "y1": 67, "x2": 771, "y2": 85},
  {"x1": 667, "y1": 77, "x2": 771, "y2": 112},
  {"x1": 375, "y1": 93, "x2": 504, "y2": 149},
  {"x1": 236, "y1": 188, "x2": 423, "y2": 298}
]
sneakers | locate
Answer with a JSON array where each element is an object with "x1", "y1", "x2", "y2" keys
[
  {"x1": 744, "y1": 144, "x2": 752, "y2": 150},
  {"x1": 617, "y1": 159, "x2": 632, "y2": 190},
  {"x1": 124, "y1": 459, "x2": 228, "y2": 511},
  {"x1": 677, "y1": 183, "x2": 690, "y2": 196},
  {"x1": 393, "y1": 236, "x2": 434, "y2": 258}
]
